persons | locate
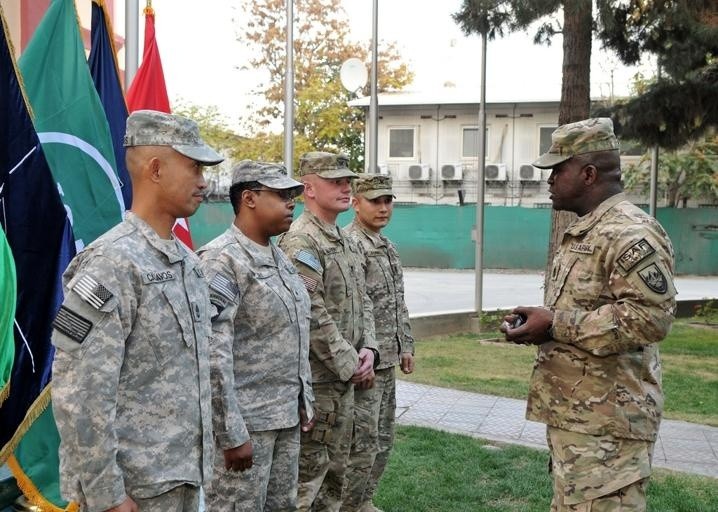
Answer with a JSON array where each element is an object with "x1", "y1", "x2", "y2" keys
[
  {"x1": 195, "y1": 159, "x2": 317, "y2": 511},
  {"x1": 274, "y1": 153, "x2": 380, "y2": 511},
  {"x1": 338, "y1": 175, "x2": 416, "y2": 512},
  {"x1": 500, "y1": 116, "x2": 679, "y2": 512},
  {"x1": 50, "y1": 111, "x2": 224, "y2": 511}
]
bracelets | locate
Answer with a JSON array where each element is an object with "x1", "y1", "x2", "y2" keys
[{"x1": 547, "y1": 319, "x2": 554, "y2": 342}]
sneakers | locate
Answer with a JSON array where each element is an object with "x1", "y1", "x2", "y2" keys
[{"x1": 359, "y1": 498, "x2": 387, "y2": 512}]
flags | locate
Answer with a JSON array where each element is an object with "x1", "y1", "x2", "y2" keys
[
  {"x1": 8, "y1": 1, "x2": 130, "y2": 509},
  {"x1": 122, "y1": 6, "x2": 196, "y2": 250},
  {"x1": 84, "y1": 2, "x2": 132, "y2": 212},
  {"x1": 0, "y1": 7, "x2": 83, "y2": 470}
]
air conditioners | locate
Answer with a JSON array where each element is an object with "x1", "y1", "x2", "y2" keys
[
  {"x1": 439, "y1": 162, "x2": 463, "y2": 180},
  {"x1": 407, "y1": 164, "x2": 430, "y2": 181},
  {"x1": 377, "y1": 163, "x2": 389, "y2": 174},
  {"x1": 518, "y1": 163, "x2": 542, "y2": 181},
  {"x1": 484, "y1": 163, "x2": 507, "y2": 181}
]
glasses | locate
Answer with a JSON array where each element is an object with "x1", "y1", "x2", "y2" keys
[{"x1": 249, "y1": 188, "x2": 297, "y2": 199}]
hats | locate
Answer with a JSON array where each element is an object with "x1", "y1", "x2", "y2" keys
[
  {"x1": 122, "y1": 109, "x2": 224, "y2": 166},
  {"x1": 298, "y1": 150, "x2": 359, "y2": 180},
  {"x1": 531, "y1": 116, "x2": 619, "y2": 170},
  {"x1": 231, "y1": 159, "x2": 304, "y2": 198},
  {"x1": 351, "y1": 172, "x2": 397, "y2": 201}
]
